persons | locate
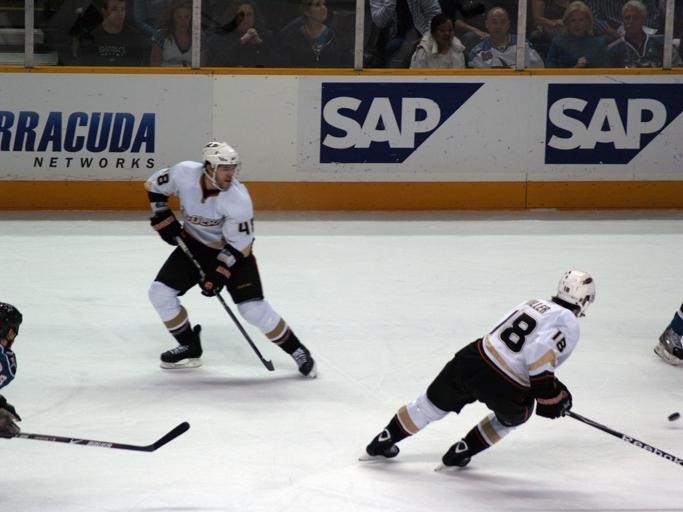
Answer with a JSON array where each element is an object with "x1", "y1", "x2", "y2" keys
[
  {"x1": 658, "y1": 304, "x2": 683, "y2": 359},
  {"x1": 367, "y1": 269, "x2": 594, "y2": 466},
  {"x1": 34, "y1": 1, "x2": 683, "y2": 68},
  {"x1": 144, "y1": 143, "x2": 312, "y2": 374},
  {"x1": 0, "y1": 302, "x2": 23, "y2": 437}
]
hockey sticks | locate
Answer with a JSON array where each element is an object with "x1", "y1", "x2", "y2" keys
[
  {"x1": 15, "y1": 422, "x2": 190, "y2": 451},
  {"x1": 175, "y1": 235, "x2": 275, "y2": 373}
]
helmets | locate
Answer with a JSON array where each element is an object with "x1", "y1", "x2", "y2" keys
[
  {"x1": 556, "y1": 269, "x2": 595, "y2": 317},
  {"x1": 202, "y1": 141, "x2": 241, "y2": 192},
  {"x1": 0, "y1": 301, "x2": 22, "y2": 338}
]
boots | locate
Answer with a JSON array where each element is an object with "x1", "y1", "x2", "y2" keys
[
  {"x1": 291, "y1": 345, "x2": 312, "y2": 375},
  {"x1": 367, "y1": 428, "x2": 400, "y2": 457},
  {"x1": 161, "y1": 324, "x2": 202, "y2": 363},
  {"x1": 659, "y1": 327, "x2": 683, "y2": 359},
  {"x1": 442, "y1": 439, "x2": 471, "y2": 466}
]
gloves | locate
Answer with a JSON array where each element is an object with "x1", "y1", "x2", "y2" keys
[
  {"x1": 0, "y1": 395, "x2": 22, "y2": 438},
  {"x1": 199, "y1": 261, "x2": 233, "y2": 297},
  {"x1": 151, "y1": 209, "x2": 185, "y2": 245},
  {"x1": 536, "y1": 378, "x2": 572, "y2": 419}
]
